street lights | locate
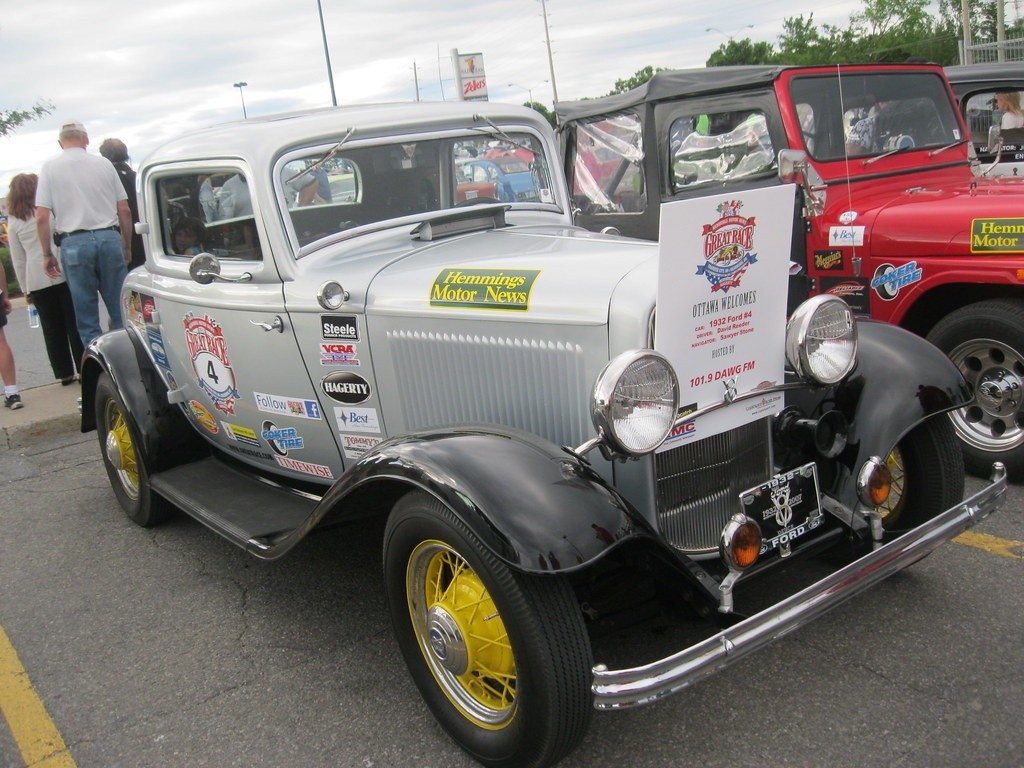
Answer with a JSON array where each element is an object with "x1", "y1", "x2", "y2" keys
[{"x1": 234, "y1": 81, "x2": 248, "y2": 119}]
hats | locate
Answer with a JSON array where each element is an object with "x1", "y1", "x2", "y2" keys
[{"x1": 60, "y1": 122, "x2": 87, "y2": 134}]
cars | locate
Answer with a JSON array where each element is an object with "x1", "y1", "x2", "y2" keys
[{"x1": 76, "y1": 102, "x2": 1008, "y2": 768}]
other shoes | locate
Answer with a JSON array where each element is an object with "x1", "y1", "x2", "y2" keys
[
  {"x1": 3, "y1": 394, "x2": 24, "y2": 410},
  {"x1": 75, "y1": 373, "x2": 82, "y2": 383},
  {"x1": 61, "y1": 371, "x2": 73, "y2": 385}
]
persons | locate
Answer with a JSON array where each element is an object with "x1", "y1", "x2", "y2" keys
[
  {"x1": 846, "y1": 96, "x2": 947, "y2": 157},
  {"x1": 802, "y1": 112, "x2": 816, "y2": 154},
  {"x1": 36, "y1": 121, "x2": 133, "y2": 349},
  {"x1": 309, "y1": 159, "x2": 333, "y2": 205},
  {"x1": 0, "y1": 262, "x2": 24, "y2": 410},
  {"x1": 220, "y1": 169, "x2": 316, "y2": 219},
  {"x1": 996, "y1": 92, "x2": 1024, "y2": 153},
  {"x1": 467, "y1": 138, "x2": 527, "y2": 159},
  {"x1": 6, "y1": 173, "x2": 86, "y2": 385},
  {"x1": 100, "y1": 138, "x2": 146, "y2": 296}
]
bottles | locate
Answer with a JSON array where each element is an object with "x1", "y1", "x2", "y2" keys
[{"x1": 27, "y1": 304, "x2": 39, "y2": 328}]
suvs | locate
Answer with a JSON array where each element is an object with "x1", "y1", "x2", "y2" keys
[{"x1": 551, "y1": 61, "x2": 1024, "y2": 485}]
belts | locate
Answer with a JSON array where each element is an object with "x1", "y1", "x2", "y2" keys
[{"x1": 57, "y1": 225, "x2": 120, "y2": 241}]
